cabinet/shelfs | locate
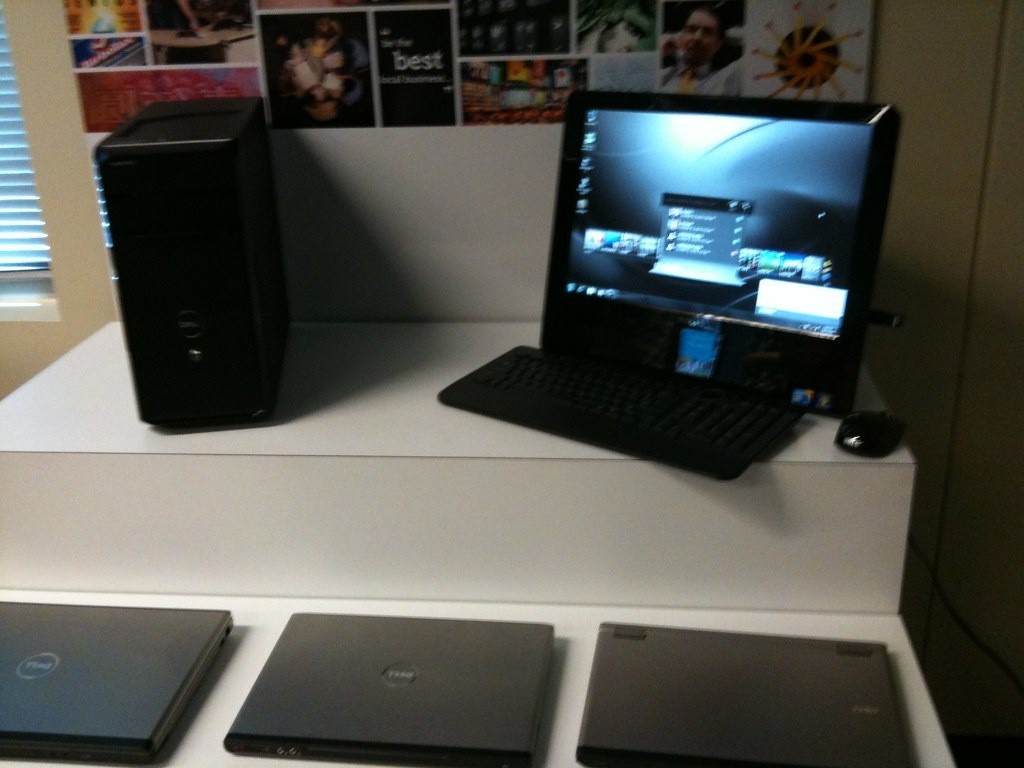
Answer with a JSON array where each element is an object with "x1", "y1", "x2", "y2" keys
[{"x1": 0, "y1": 317, "x2": 959, "y2": 768}]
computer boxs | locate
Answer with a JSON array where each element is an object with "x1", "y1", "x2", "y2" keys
[{"x1": 91, "y1": 97, "x2": 295, "y2": 429}]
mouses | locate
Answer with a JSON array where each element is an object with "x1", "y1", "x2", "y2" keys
[{"x1": 834, "y1": 410, "x2": 905, "y2": 459}]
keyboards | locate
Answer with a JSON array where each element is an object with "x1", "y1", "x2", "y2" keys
[{"x1": 437, "y1": 345, "x2": 806, "y2": 481}]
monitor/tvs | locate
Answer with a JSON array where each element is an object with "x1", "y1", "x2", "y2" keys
[{"x1": 548, "y1": 88, "x2": 902, "y2": 379}]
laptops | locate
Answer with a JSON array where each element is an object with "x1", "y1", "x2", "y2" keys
[
  {"x1": 224, "y1": 612, "x2": 554, "y2": 768},
  {"x1": 577, "y1": 624, "x2": 913, "y2": 768},
  {"x1": 0, "y1": 602, "x2": 234, "y2": 763}
]
